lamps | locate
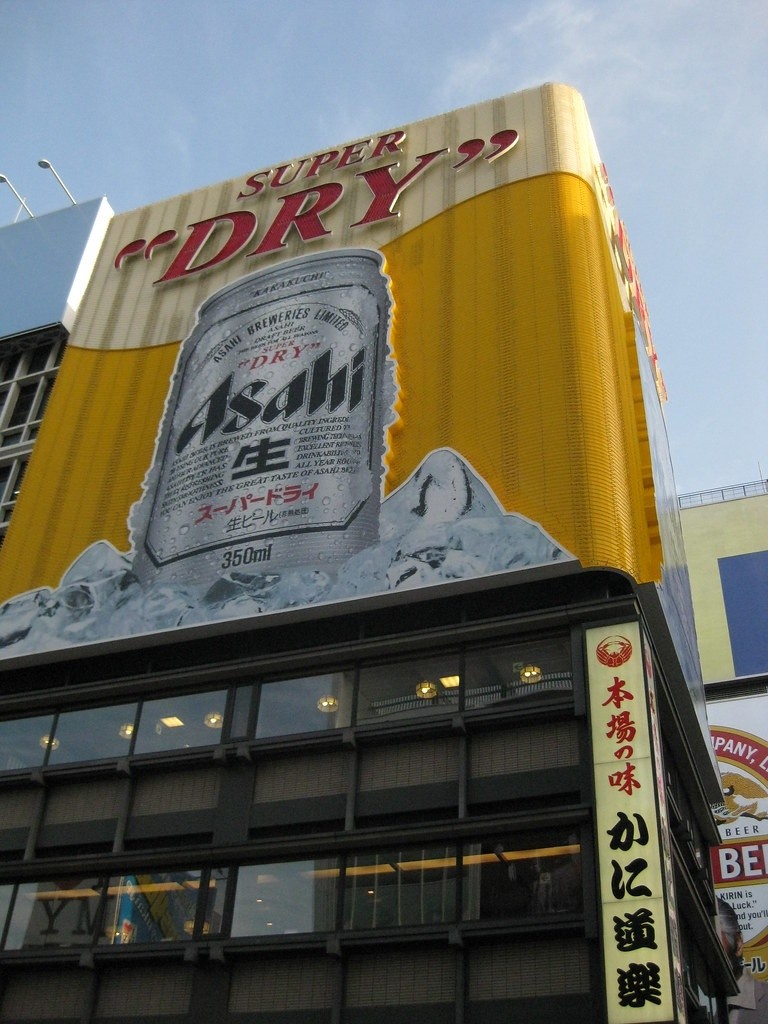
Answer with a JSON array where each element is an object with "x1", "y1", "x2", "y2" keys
[
  {"x1": 118, "y1": 723, "x2": 135, "y2": 739},
  {"x1": 204, "y1": 713, "x2": 224, "y2": 728},
  {"x1": 40, "y1": 732, "x2": 60, "y2": 750},
  {"x1": 317, "y1": 694, "x2": 339, "y2": 713},
  {"x1": 416, "y1": 680, "x2": 438, "y2": 699},
  {"x1": 185, "y1": 919, "x2": 209, "y2": 936},
  {"x1": 520, "y1": 663, "x2": 542, "y2": 682}
]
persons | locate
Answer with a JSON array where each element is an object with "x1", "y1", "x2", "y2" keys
[{"x1": 717, "y1": 897, "x2": 768, "y2": 1024}]
